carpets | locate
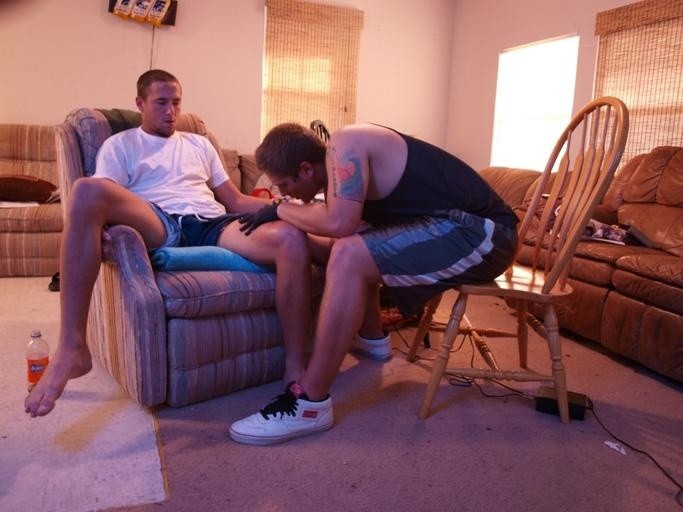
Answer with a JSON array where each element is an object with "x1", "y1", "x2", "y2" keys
[{"x1": 91, "y1": 288, "x2": 683, "y2": 511}]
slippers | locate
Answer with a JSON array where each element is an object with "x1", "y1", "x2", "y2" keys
[{"x1": 49, "y1": 272, "x2": 60, "y2": 292}]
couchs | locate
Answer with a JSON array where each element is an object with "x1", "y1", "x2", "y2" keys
[{"x1": 480, "y1": 145, "x2": 683, "y2": 387}]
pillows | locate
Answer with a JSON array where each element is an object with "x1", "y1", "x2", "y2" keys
[{"x1": 0, "y1": 174, "x2": 58, "y2": 204}]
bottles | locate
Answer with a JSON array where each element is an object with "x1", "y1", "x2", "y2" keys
[{"x1": 25, "y1": 330, "x2": 50, "y2": 393}]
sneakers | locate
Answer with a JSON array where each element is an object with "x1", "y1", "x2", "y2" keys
[
  {"x1": 229, "y1": 380, "x2": 334, "y2": 445},
  {"x1": 349, "y1": 328, "x2": 395, "y2": 362}
]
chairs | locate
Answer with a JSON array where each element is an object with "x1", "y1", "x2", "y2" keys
[
  {"x1": 407, "y1": 95, "x2": 630, "y2": 426},
  {"x1": 0, "y1": 124, "x2": 60, "y2": 277},
  {"x1": 55, "y1": 104, "x2": 314, "y2": 410}
]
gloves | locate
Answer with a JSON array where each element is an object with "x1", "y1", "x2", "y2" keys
[{"x1": 238, "y1": 204, "x2": 280, "y2": 236}]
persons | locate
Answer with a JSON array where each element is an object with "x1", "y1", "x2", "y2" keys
[
  {"x1": 22, "y1": 69, "x2": 315, "y2": 419},
  {"x1": 227, "y1": 121, "x2": 520, "y2": 449}
]
